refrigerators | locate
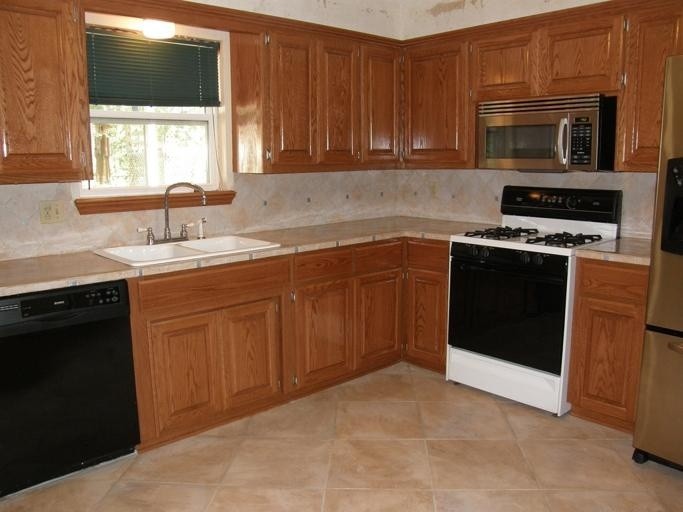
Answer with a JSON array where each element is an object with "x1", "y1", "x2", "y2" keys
[{"x1": 629, "y1": 53, "x2": 682, "y2": 473}]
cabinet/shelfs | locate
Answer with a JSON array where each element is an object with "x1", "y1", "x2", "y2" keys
[
  {"x1": 401, "y1": 25, "x2": 475, "y2": 171},
  {"x1": 475, "y1": 0, "x2": 621, "y2": 102},
  {"x1": 290, "y1": 237, "x2": 403, "y2": 400},
  {"x1": 127, "y1": 254, "x2": 289, "y2": 452},
  {"x1": 566, "y1": 254, "x2": 651, "y2": 432},
  {"x1": 0, "y1": 0, "x2": 94, "y2": 186},
  {"x1": 620, "y1": 1, "x2": 683, "y2": 171},
  {"x1": 402, "y1": 236, "x2": 450, "y2": 377},
  {"x1": 316, "y1": 26, "x2": 401, "y2": 174},
  {"x1": 230, "y1": 20, "x2": 315, "y2": 176}
]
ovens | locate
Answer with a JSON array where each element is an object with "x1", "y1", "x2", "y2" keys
[{"x1": 443, "y1": 256, "x2": 572, "y2": 420}]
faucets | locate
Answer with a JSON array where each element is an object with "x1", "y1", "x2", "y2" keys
[{"x1": 164, "y1": 181, "x2": 207, "y2": 240}]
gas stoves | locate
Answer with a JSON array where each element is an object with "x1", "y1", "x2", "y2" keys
[{"x1": 447, "y1": 215, "x2": 618, "y2": 271}]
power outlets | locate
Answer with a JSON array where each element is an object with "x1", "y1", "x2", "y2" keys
[{"x1": 39, "y1": 200, "x2": 54, "y2": 224}]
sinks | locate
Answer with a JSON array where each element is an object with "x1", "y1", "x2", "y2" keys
[
  {"x1": 95, "y1": 243, "x2": 204, "y2": 267},
  {"x1": 179, "y1": 236, "x2": 279, "y2": 253}
]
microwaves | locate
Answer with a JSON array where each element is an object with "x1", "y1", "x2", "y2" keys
[{"x1": 476, "y1": 93, "x2": 614, "y2": 175}]
